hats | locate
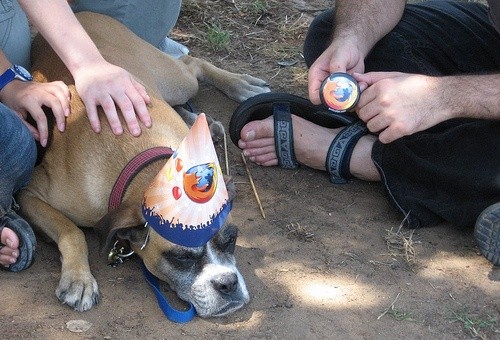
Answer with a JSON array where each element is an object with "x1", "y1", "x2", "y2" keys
[{"x1": 140, "y1": 112, "x2": 233, "y2": 248}]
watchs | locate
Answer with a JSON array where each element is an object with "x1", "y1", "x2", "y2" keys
[{"x1": 0, "y1": 63, "x2": 34, "y2": 91}]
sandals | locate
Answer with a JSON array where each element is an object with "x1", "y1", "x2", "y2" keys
[
  {"x1": 0, "y1": 205, "x2": 37, "y2": 273},
  {"x1": 475, "y1": 202, "x2": 500, "y2": 266},
  {"x1": 229, "y1": 93, "x2": 380, "y2": 184}
]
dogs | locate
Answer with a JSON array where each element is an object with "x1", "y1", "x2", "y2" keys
[{"x1": 12, "y1": 11, "x2": 272, "y2": 319}]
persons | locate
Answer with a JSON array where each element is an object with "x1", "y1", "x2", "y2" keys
[
  {"x1": 230, "y1": 0, "x2": 499, "y2": 233},
  {"x1": 0, "y1": 0, "x2": 188, "y2": 136},
  {"x1": 0, "y1": 47, "x2": 72, "y2": 275},
  {"x1": 472, "y1": 202, "x2": 500, "y2": 267}
]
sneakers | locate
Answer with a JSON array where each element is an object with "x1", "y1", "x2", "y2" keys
[{"x1": 164, "y1": 38, "x2": 191, "y2": 58}]
